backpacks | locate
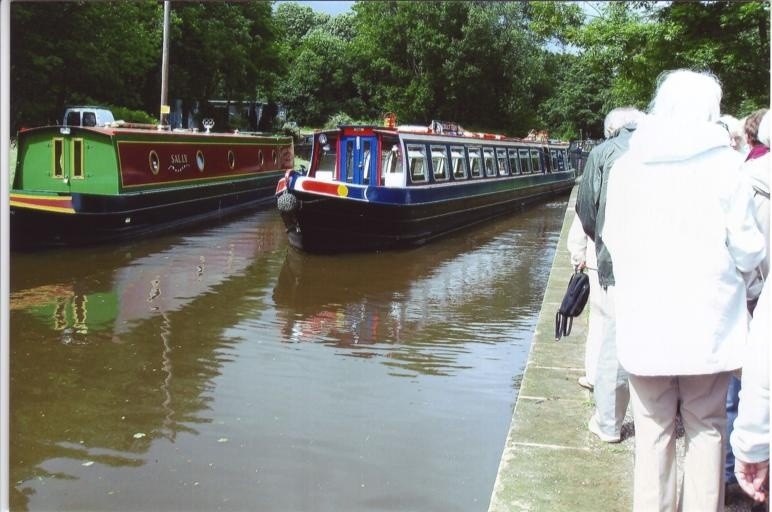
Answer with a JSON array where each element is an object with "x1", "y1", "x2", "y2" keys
[{"x1": 554, "y1": 263, "x2": 590, "y2": 340}]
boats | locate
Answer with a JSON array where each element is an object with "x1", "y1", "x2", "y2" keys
[
  {"x1": 270, "y1": 119, "x2": 583, "y2": 257},
  {"x1": 270, "y1": 194, "x2": 572, "y2": 352},
  {"x1": 9, "y1": 203, "x2": 288, "y2": 346},
  {"x1": 9, "y1": 101, "x2": 304, "y2": 246}
]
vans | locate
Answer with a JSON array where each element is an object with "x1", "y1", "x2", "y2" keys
[{"x1": 61, "y1": 106, "x2": 118, "y2": 128}]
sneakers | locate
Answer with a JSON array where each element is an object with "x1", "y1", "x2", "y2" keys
[
  {"x1": 577, "y1": 376, "x2": 593, "y2": 390},
  {"x1": 587, "y1": 413, "x2": 621, "y2": 443}
]
